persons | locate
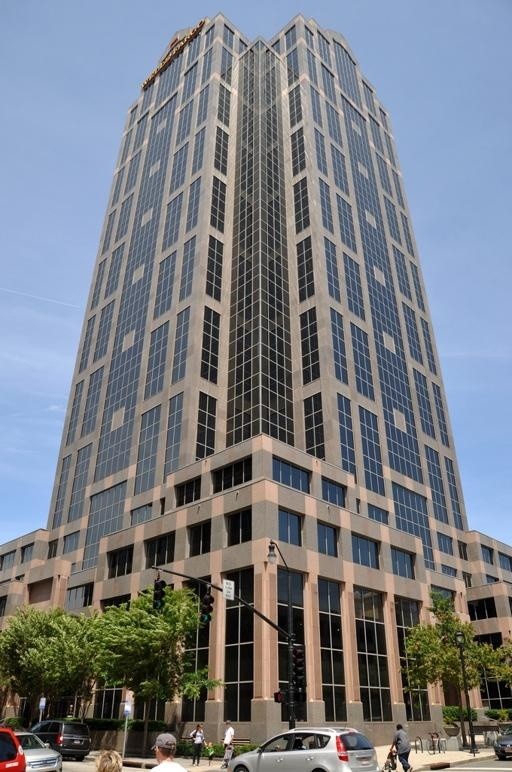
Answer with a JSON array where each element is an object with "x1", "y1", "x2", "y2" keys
[
  {"x1": 189, "y1": 724, "x2": 207, "y2": 767},
  {"x1": 205, "y1": 742, "x2": 217, "y2": 767},
  {"x1": 274, "y1": 739, "x2": 303, "y2": 752},
  {"x1": 96, "y1": 750, "x2": 123, "y2": 772},
  {"x1": 148, "y1": 733, "x2": 187, "y2": 772},
  {"x1": 390, "y1": 724, "x2": 413, "y2": 772},
  {"x1": 220, "y1": 719, "x2": 235, "y2": 769}
]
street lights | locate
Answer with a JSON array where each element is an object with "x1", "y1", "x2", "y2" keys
[
  {"x1": 453, "y1": 629, "x2": 478, "y2": 753},
  {"x1": 267, "y1": 538, "x2": 297, "y2": 730}
]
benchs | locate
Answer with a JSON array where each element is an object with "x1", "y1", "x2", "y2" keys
[{"x1": 468, "y1": 726, "x2": 499, "y2": 743}]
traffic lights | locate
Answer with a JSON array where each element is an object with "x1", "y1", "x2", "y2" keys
[
  {"x1": 292, "y1": 646, "x2": 306, "y2": 702},
  {"x1": 152, "y1": 578, "x2": 166, "y2": 610},
  {"x1": 200, "y1": 593, "x2": 214, "y2": 624},
  {"x1": 274, "y1": 691, "x2": 288, "y2": 703}
]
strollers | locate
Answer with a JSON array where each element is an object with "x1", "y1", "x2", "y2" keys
[{"x1": 382, "y1": 749, "x2": 398, "y2": 771}]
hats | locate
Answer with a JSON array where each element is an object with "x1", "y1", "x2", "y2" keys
[{"x1": 151, "y1": 733, "x2": 177, "y2": 750}]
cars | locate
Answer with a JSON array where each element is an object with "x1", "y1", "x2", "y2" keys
[
  {"x1": 227, "y1": 726, "x2": 379, "y2": 772},
  {"x1": 1, "y1": 727, "x2": 26, "y2": 771},
  {"x1": 493, "y1": 725, "x2": 511, "y2": 760},
  {"x1": 14, "y1": 730, "x2": 64, "y2": 772}
]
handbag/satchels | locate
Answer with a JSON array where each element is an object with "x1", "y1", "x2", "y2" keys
[{"x1": 191, "y1": 732, "x2": 196, "y2": 743}]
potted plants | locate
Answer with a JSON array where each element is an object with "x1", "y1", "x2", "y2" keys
[
  {"x1": 443, "y1": 716, "x2": 460, "y2": 736},
  {"x1": 497, "y1": 709, "x2": 512, "y2": 728}
]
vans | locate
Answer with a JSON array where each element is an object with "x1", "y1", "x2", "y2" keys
[{"x1": 30, "y1": 719, "x2": 92, "y2": 761}]
